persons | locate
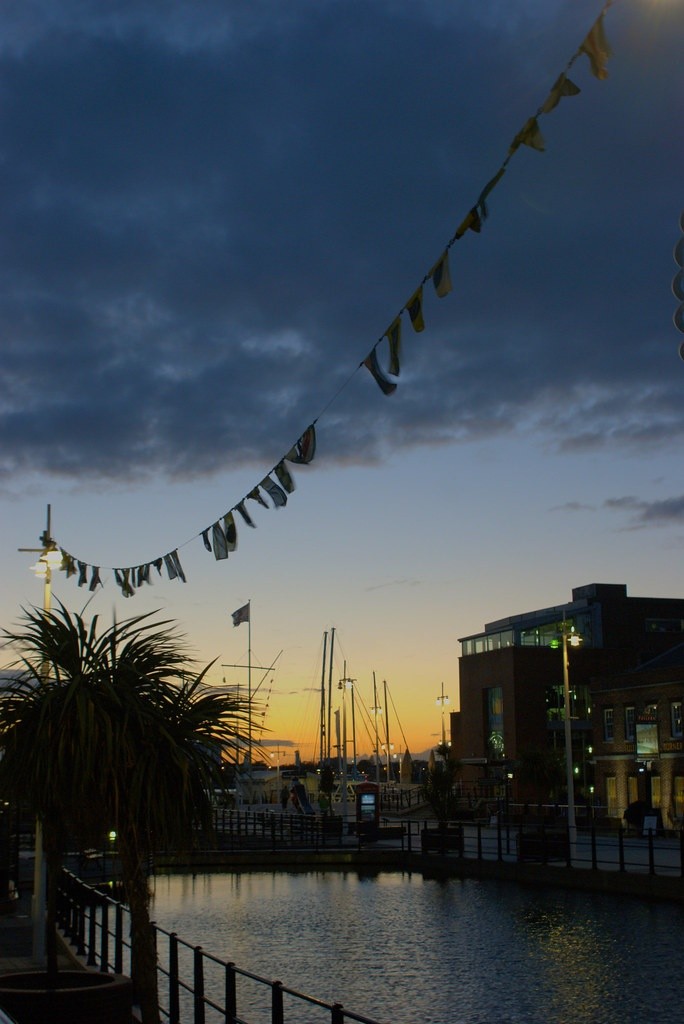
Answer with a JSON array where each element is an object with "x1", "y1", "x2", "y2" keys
[
  {"x1": 318, "y1": 795, "x2": 329, "y2": 814},
  {"x1": 228, "y1": 794, "x2": 236, "y2": 810},
  {"x1": 280, "y1": 786, "x2": 290, "y2": 809}
]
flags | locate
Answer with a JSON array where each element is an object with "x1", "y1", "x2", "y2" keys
[
  {"x1": 54, "y1": 12, "x2": 612, "y2": 597},
  {"x1": 232, "y1": 602, "x2": 250, "y2": 627}
]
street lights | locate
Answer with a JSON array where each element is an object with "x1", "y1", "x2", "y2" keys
[
  {"x1": 338, "y1": 679, "x2": 354, "y2": 835},
  {"x1": 437, "y1": 696, "x2": 450, "y2": 770},
  {"x1": 270, "y1": 751, "x2": 286, "y2": 803},
  {"x1": 550, "y1": 630, "x2": 584, "y2": 842}
]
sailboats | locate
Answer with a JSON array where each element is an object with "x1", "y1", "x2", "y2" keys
[{"x1": 367, "y1": 679, "x2": 421, "y2": 808}]
